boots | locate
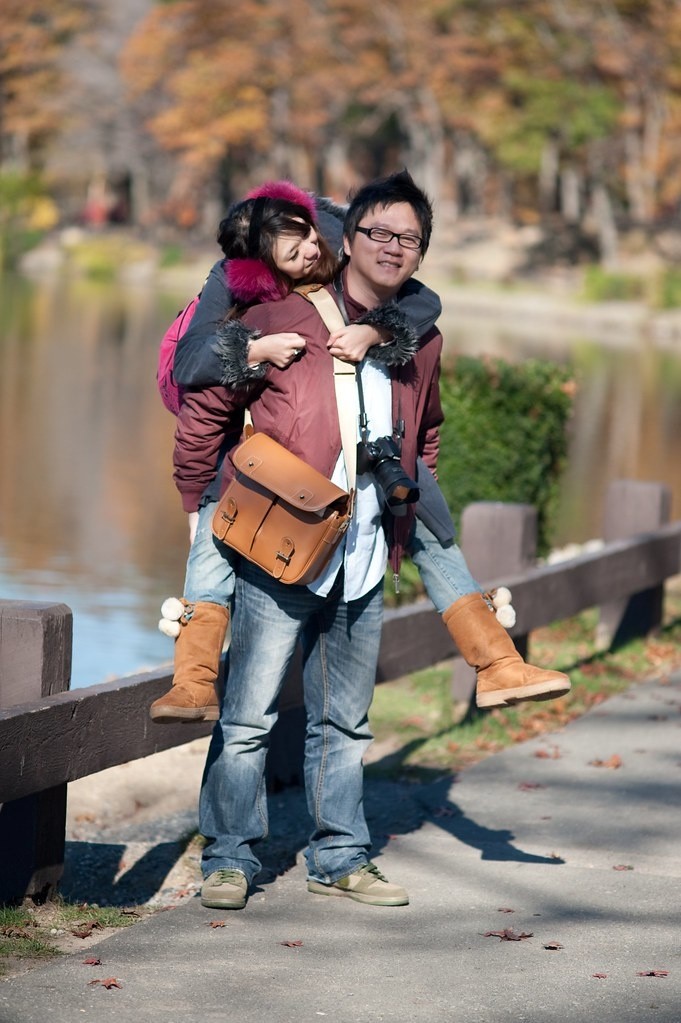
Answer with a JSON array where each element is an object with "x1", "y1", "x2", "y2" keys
[
  {"x1": 149, "y1": 597, "x2": 230, "y2": 723},
  {"x1": 442, "y1": 588, "x2": 571, "y2": 710}
]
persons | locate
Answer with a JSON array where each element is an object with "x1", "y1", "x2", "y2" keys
[
  {"x1": 171, "y1": 169, "x2": 444, "y2": 909},
  {"x1": 149, "y1": 182, "x2": 571, "y2": 722}
]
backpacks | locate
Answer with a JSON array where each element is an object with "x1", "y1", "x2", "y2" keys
[{"x1": 157, "y1": 295, "x2": 200, "y2": 418}]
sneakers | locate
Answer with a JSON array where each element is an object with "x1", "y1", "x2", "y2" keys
[
  {"x1": 201, "y1": 869, "x2": 247, "y2": 907},
  {"x1": 309, "y1": 864, "x2": 409, "y2": 906}
]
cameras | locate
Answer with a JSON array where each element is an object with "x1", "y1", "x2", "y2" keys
[{"x1": 356, "y1": 436, "x2": 423, "y2": 509}]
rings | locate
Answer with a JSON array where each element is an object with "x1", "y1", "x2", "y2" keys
[{"x1": 294, "y1": 350, "x2": 298, "y2": 355}]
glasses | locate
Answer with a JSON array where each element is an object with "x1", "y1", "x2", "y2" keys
[{"x1": 355, "y1": 226, "x2": 424, "y2": 249}]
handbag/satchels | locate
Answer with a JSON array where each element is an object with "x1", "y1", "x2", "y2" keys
[{"x1": 210, "y1": 431, "x2": 351, "y2": 586}]
